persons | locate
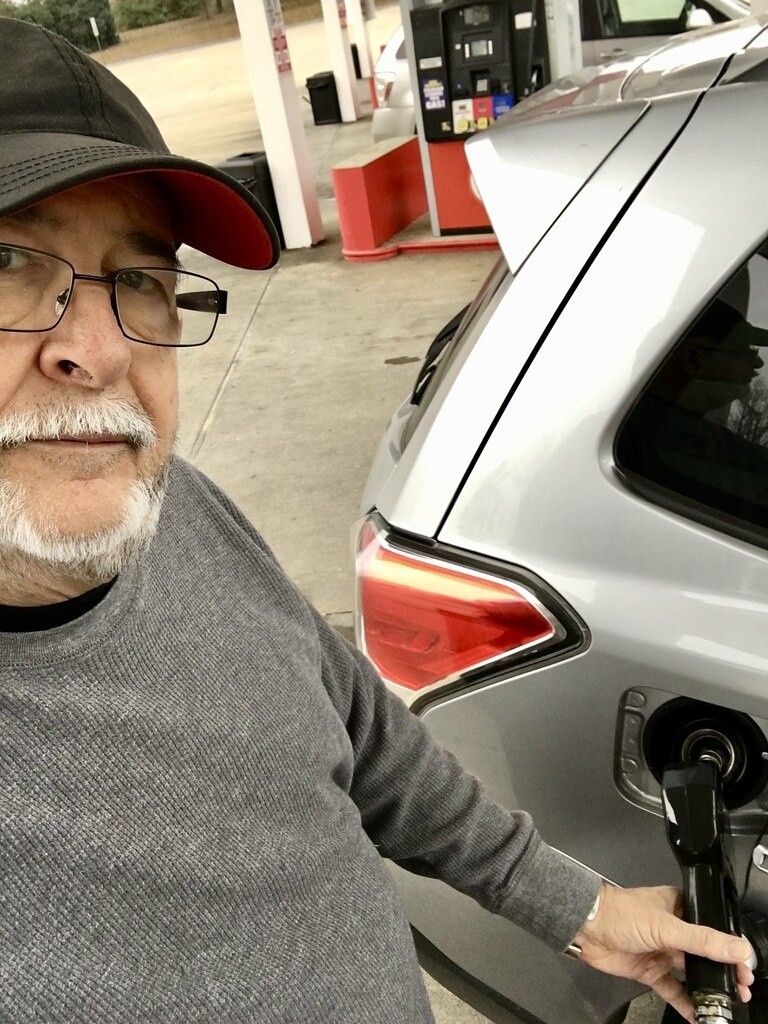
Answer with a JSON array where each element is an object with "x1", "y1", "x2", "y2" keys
[{"x1": 0, "y1": 14, "x2": 757, "y2": 1024}]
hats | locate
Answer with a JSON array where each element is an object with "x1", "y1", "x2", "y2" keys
[{"x1": 0, "y1": 15, "x2": 280, "y2": 271}]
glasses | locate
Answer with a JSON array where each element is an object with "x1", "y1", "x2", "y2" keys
[{"x1": 1, "y1": 243, "x2": 227, "y2": 347}]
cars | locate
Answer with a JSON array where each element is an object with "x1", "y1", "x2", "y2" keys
[
  {"x1": 577, "y1": 0, "x2": 753, "y2": 72},
  {"x1": 352, "y1": 2, "x2": 768, "y2": 1023}
]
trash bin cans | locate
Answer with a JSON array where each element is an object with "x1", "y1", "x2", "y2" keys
[
  {"x1": 215, "y1": 150, "x2": 285, "y2": 247},
  {"x1": 305, "y1": 70, "x2": 343, "y2": 125}
]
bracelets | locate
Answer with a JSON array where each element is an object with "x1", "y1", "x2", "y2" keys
[{"x1": 559, "y1": 887, "x2": 600, "y2": 961}]
